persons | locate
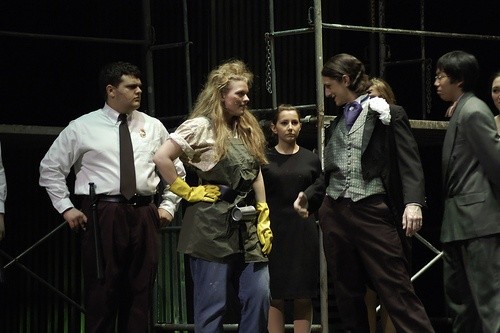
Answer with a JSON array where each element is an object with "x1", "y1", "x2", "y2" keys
[
  {"x1": 39, "y1": 62, "x2": 186, "y2": 333},
  {"x1": 435, "y1": 51, "x2": 500, "y2": 333},
  {"x1": 492, "y1": 73, "x2": 500, "y2": 134},
  {"x1": 294, "y1": 54, "x2": 429, "y2": 333},
  {"x1": 153, "y1": 64, "x2": 272, "y2": 333},
  {"x1": 365, "y1": 78, "x2": 396, "y2": 333},
  {"x1": 262, "y1": 105, "x2": 323, "y2": 333}
]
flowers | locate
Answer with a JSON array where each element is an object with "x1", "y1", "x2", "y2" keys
[{"x1": 369, "y1": 95, "x2": 391, "y2": 125}]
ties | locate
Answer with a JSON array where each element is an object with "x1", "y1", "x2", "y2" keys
[{"x1": 117, "y1": 113, "x2": 136, "y2": 200}]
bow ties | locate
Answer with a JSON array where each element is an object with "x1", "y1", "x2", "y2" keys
[{"x1": 445, "y1": 102, "x2": 458, "y2": 118}]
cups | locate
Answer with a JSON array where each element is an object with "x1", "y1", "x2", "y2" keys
[{"x1": 231, "y1": 200, "x2": 256, "y2": 221}]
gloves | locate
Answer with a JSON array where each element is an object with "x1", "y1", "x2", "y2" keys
[
  {"x1": 169, "y1": 177, "x2": 222, "y2": 204},
  {"x1": 255, "y1": 201, "x2": 273, "y2": 256}
]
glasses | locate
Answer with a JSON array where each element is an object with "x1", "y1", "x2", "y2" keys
[{"x1": 434, "y1": 75, "x2": 451, "y2": 80}]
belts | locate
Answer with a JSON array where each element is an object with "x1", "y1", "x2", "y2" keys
[
  {"x1": 218, "y1": 184, "x2": 254, "y2": 207},
  {"x1": 80, "y1": 192, "x2": 154, "y2": 206}
]
